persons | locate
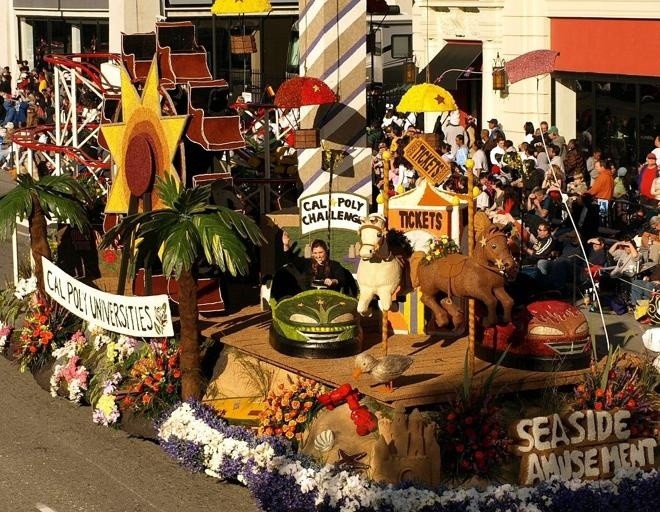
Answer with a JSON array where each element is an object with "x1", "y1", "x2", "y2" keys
[
  {"x1": 367, "y1": 90, "x2": 660, "y2": 293},
  {"x1": 0, "y1": 35, "x2": 109, "y2": 176},
  {"x1": 281, "y1": 232, "x2": 354, "y2": 292},
  {"x1": 230, "y1": 95, "x2": 248, "y2": 111}
]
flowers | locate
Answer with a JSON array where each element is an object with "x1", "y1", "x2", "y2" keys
[
  {"x1": 156, "y1": 331, "x2": 660, "y2": 512},
  {"x1": 0, "y1": 274, "x2": 182, "y2": 429}
]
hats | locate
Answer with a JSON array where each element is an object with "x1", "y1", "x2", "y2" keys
[
  {"x1": 618, "y1": 167, "x2": 628, "y2": 177},
  {"x1": 545, "y1": 126, "x2": 558, "y2": 134},
  {"x1": 648, "y1": 153, "x2": 657, "y2": 159},
  {"x1": 487, "y1": 119, "x2": 497, "y2": 125}
]
